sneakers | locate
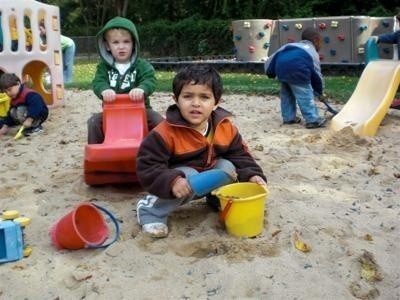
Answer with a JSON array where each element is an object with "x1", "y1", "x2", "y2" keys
[
  {"x1": 284, "y1": 115, "x2": 301, "y2": 126},
  {"x1": 141, "y1": 223, "x2": 169, "y2": 239},
  {"x1": 305, "y1": 116, "x2": 329, "y2": 129},
  {"x1": 24, "y1": 126, "x2": 45, "y2": 137}
]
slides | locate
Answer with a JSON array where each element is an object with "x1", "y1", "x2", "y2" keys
[{"x1": 330, "y1": 59, "x2": 399, "y2": 139}]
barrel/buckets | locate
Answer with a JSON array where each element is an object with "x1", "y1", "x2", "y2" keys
[
  {"x1": 211, "y1": 183, "x2": 269, "y2": 238},
  {"x1": 50, "y1": 202, "x2": 119, "y2": 249}
]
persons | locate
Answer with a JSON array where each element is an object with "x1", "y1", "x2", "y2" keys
[
  {"x1": 264, "y1": 29, "x2": 327, "y2": 129},
  {"x1": 39, "y1": 22, "x2": 76, "y2": 84},
  {"x1": 372, "y1": 13, "x2": 400, "y2": 61},
  {"x1": 137, "y1": 64, "x2": 268, "y2": 237},
  {"x1": 86, "y1": 17, "x2": 163, "y2": 142},
  {"x1": 0, "y1": 72, "x2": 48, "y2": 136},
  {"x1": 11, "y1": 16, "x2": 32, "y2": 51}
]
